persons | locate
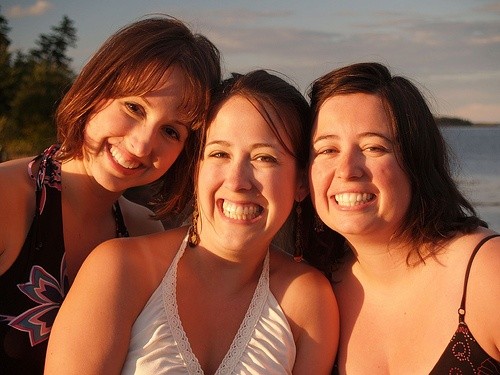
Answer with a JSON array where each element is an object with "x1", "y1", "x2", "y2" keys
[
  {"x1": 43, "y1": 69, "x2": 341, "y2": 375},
  {"x1": 1, "y1": 12, "x2": 223, "y2": 372},
  {"x1": 308, "y1": 62, "x2": 500, "y2": 375}
]
